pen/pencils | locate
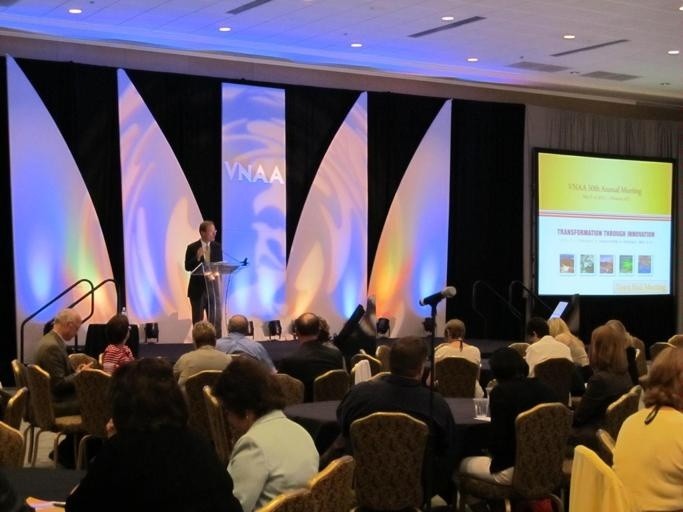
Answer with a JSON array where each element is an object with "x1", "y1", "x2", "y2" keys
[{"x1": 32, "y1": 502, "x2": 53, "y2": 508}]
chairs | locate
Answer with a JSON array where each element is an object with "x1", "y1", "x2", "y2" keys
[
  {"x1": 348, "y1": 409, "x2": 432, "y2": 512},
  {"x1": 487, "y1": 331, "x2": 683, "y2": 467},
  {"x1": 565, "y1": 440, "x2": 633, "y2": 511},
  {"x1": 183, "y1": 368, "x2": 230, "y2": 464},
  {"x1": 448, "y1": 400, "x2": 577, "y2": 511},
  {"x1": 275, "y1": 343, "x2": 384, "y2": 408},
  {"x1": 0, "y1": 353, "x2": 112, "y2": 469},
  {"x1": 253, "y1": 454, "x2": 355, "y2": 512}
]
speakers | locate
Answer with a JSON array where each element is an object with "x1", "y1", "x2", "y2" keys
[{"x1": 85, "y1": 324, "x2": 139, "y2": 358}]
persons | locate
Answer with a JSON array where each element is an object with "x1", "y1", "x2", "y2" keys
[{"x1": 184, "y1": 220, "x2": 224, "y2": 338}]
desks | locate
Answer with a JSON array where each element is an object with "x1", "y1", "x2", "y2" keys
[{"x1": 282, "y1": 395, "x2": 494, "y2": 500}]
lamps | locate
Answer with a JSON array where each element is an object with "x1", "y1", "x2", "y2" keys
[
  {"x1": 266, "y1": 320, "x2": 283, "y2": 340},
  {"x1": 142, "y1": 320, "x2": 160, "y2": 343},
  {"x1": 419, "y1": 315, "x2": 437, "y2": 337},
  {"x1": 245, "y1": 320, "x2": 255, "y2": 343},
  {"x1": 375, "y1": 316, "x2": 391, "y2": 340}
]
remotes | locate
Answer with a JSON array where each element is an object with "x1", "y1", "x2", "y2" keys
[{"x1": 419, "y1": 286, "x2": 457, "y2": 306}]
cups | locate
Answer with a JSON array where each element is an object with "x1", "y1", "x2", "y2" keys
[{"x1": 472, "y1": 397, "x2": 490, "y2": 418}]
